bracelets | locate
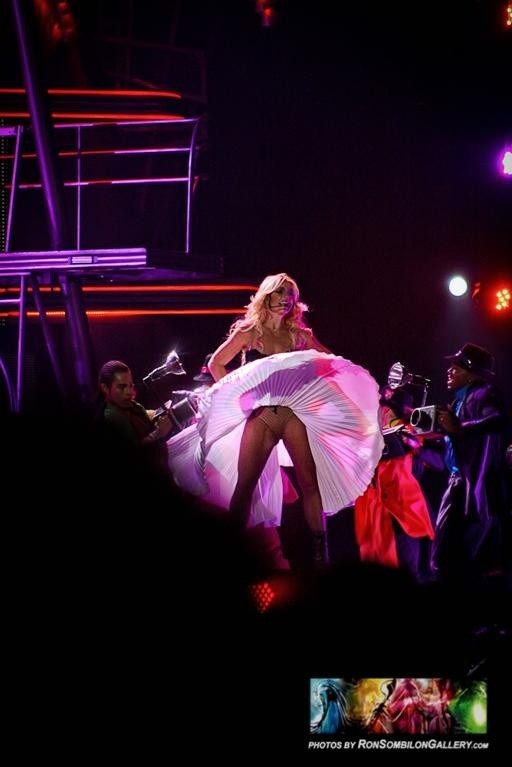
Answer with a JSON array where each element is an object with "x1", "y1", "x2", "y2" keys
[{"x1": 147, "y1": 430, "x2": 161, "y2": 441}]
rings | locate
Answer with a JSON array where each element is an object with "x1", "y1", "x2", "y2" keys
[{"x1": 440, "y1": 415, "x2": 444, "y2": 422}]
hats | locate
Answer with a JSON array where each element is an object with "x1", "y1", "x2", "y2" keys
[{"x1": 443, "y1": 343, "x2": 494, "y2": 380}]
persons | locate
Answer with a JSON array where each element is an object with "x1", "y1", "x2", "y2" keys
[
  {"x1": 193, "y1": 354, "x2": 299, "y2": 572},
  {"x1": 401, "y1": 343, "x2": 500, "y2": 571},
  {"x1": 98, "y1": 358, "x2": 174, "y2": 452},
  {"x1": 206, "y1": 271, "x2": 336, "y2": 584},
  {"x1": 354, "y1": 384, "x2": 434, "y2": 588}
]
